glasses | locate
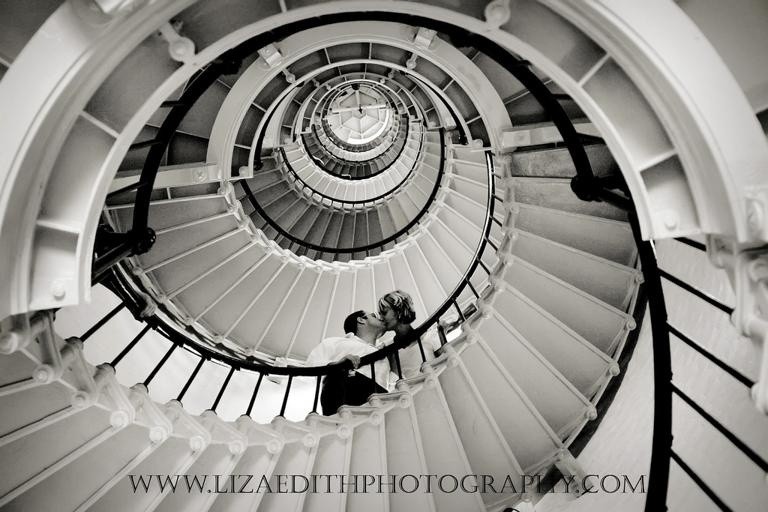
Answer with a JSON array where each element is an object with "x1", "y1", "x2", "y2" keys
[{"x1": 362, "y1": 312, "x2": 377, "y2": 318}]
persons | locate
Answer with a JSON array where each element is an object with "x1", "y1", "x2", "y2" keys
[
  {"x1": 378, "y1": 291, "x2": 437, "y2": 379},
  {"x1": 303, "y1": 310, "x2": 400, "y2": 416}
]
